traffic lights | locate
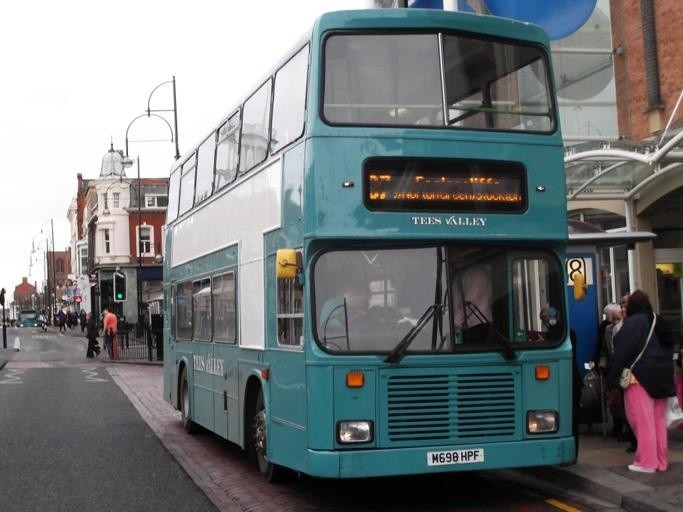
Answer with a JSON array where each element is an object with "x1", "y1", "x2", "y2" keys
[{"x1": 113, "y1": 271, "x2": 128, "y2": 303}]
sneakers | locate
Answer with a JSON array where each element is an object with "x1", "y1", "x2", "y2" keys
[{"x1": 628, "y1": 464, "x2": 657, "y2": 473}]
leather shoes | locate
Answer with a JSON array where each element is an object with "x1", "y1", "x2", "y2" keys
[{"x1": 608, "y1": 426, "x2": 635, "y2": 454}]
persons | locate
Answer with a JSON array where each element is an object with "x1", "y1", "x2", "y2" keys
[
  {"x1": 540, "y1": 303, "x2": 582, "y2": 466},
  {"x1": 675, "y1": 326, "x2": 683, "y2": 411},
  {"x1": 595, "y1": 291, "x2": 676, "y2": 475},
  {"x1": 321, "y1": 281, "x2": 396, "y2": 330},
  {"x1": 6, "y1": 303, "x2": 149, "y2": 358}
]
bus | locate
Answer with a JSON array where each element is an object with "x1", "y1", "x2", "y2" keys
[{"x1": 160, "y1": 8, "x2": 578, "y2": 487}]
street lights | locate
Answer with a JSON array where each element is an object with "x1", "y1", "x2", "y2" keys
[
  {"x1": 103, "y1": 155, "x2": 145, "y2": 338},
  {"x1": 122, "y1": 74, "x2": 181, "y2": 169}
]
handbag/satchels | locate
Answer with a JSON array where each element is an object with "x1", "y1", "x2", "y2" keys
[
  {"x1": 619, "y1": 368, "x2": 631, "y2": 389},
  {"x1": 580, "y1": 370, "x2": 600, "y2": 407}
]
